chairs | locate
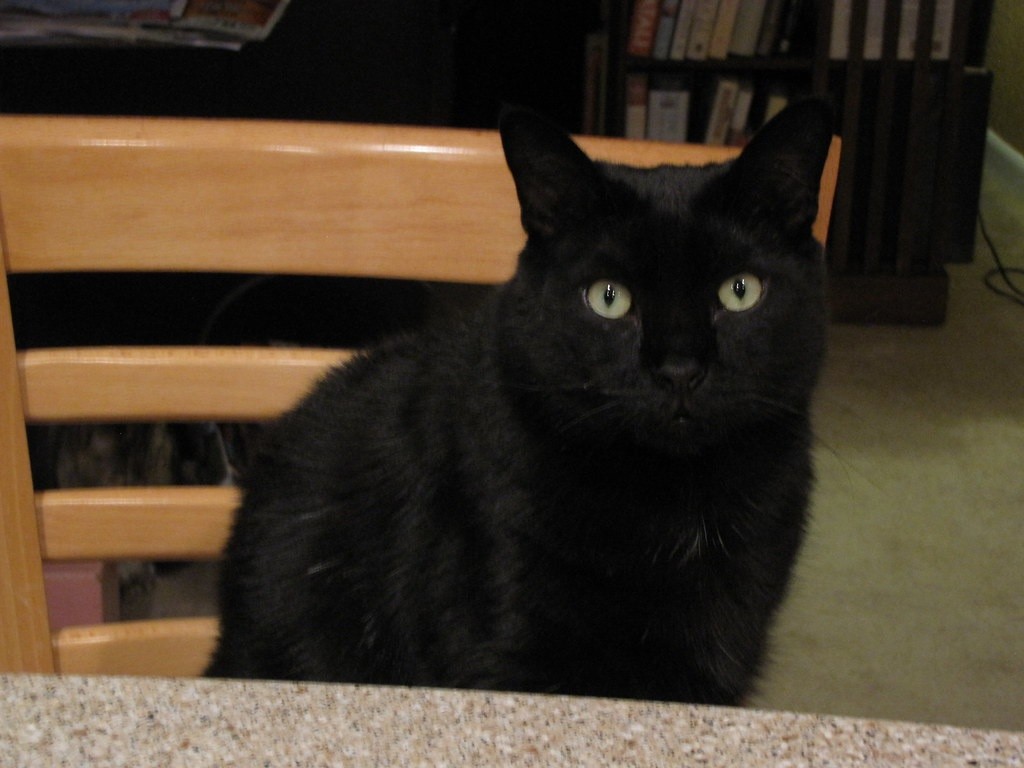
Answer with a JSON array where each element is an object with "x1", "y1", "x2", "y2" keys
[{"x1": 0, "y1": 113, "x2": 846, "y2": 675}]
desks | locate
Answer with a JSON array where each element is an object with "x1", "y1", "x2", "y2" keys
[{"x1": 0, "y1": 678, "x2": 1024, "y2": 768}]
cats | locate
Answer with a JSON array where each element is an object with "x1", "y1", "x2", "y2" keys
[{"x1": 195, "y1": 75, "x2": 841, "y2": 710}]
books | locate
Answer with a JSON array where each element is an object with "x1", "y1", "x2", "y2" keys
[
  {"x1": 829, "y1": 0, "x2": 955, "y2": 62},
  {"x1": 0, "y1": 0, "x2": 291, "y2": 52},
  {"x1": 625, "y1": 0, "x2": 801, "y2": 62},
  {"x1": 625, "y1": 72, "x2": 797, "y2": 147}
]
